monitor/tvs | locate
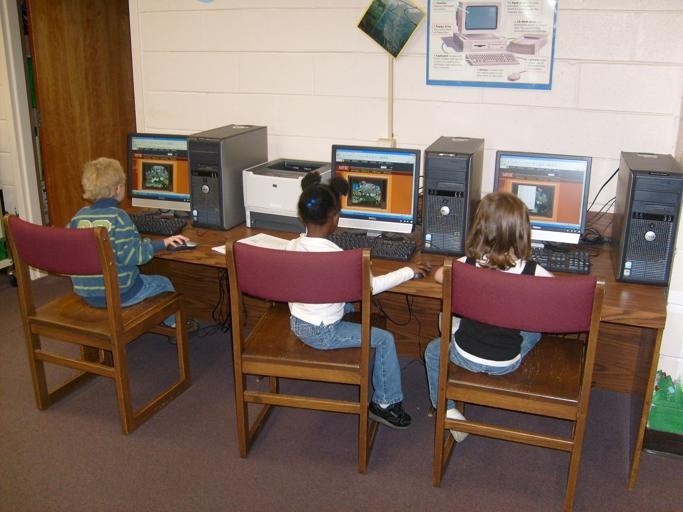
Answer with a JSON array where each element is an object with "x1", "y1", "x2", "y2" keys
[
  {"x1": 126, "y1": 133, "x2": 191, "y2": 218},
  {"x1": 453, "y1": 1, "x2": 506, "y2": 35},
  {"x1": 492, "y1": 150, "x2": 594, "y2": 249},
  {"x1": 332, "y1": 144, "x2": 423, "y2": 236}
]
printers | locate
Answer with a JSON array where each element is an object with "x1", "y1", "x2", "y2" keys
[{"x1": 241, "y1": 158, "x2": 332, "y2": 234}]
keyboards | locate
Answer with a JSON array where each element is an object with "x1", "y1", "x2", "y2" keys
[
  {"x1": 531, "y1": 247, "x2": 591, "y2": 274},
  {"x1": 464, "y1": 53, "x2": 520, "y2": 66},
  {"x1": 126, "y1": 213, "x2": 186, "y2": 235},
  {"x1": 326, "y1": 232, "x2": 417, "y2": 263}
]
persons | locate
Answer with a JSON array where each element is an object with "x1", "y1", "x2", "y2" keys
[
  {"x1": 287, "y1": 171, "x2": 432, "y2": 430},
  {"x1": 69, "y1": 156, "x2": 191, "y2": 345},
  {"x1": 424, "y1": 191, "x2": 555, "y2": 444}
]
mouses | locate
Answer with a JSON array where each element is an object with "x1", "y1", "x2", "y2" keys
[
  {"x1": 411, "y1": 267, "x2": 430, "y2": 279},
  {"x1": 508, "y1": 73, "x2": 521, "y2": 81},
  {"x1": 168, "y1": 241, "x2": 198, "y2": 251}
]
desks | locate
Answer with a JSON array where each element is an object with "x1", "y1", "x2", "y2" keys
[{"x1": 119, "y1": 196, "x2": 667, "y2": 490}]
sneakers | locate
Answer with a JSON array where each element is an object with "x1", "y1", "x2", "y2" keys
[
  {"x1": 439, "y1": 312, "x2": 461, "y2": 335},
  {"x1": 446, "y1": 409, "x2": 470, "y2": 442},
  {"x1": 169, "y1": 321, "x2": 199, "y2": 343},
  {"x1": 367, "y1": 401, "x2": 411, "y2": 430}
]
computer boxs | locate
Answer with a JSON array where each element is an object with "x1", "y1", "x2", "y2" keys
[
  {"x1": 187, "y1": 122, "x2": 267, "y2": 232},
  {"x1": 452, "y1": 32, "x2": 506, "y2": 52},
  {"x1": 421, "y1": 135, "x2": 485, "y2": 257},
  {"x1": 609, "y1": 150, "x2": 683, "y2": 287}
]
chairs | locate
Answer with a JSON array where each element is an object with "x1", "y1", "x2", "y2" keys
[
  {"x1": 431, "y1": 255, "x2": 607, "y2": 512},
  {"x1": 225, "y1": 239, "x2": 387, "y2": 475},
  {"x1": 3, "y1": 214, "x2": 193, "y2": 435}
]
